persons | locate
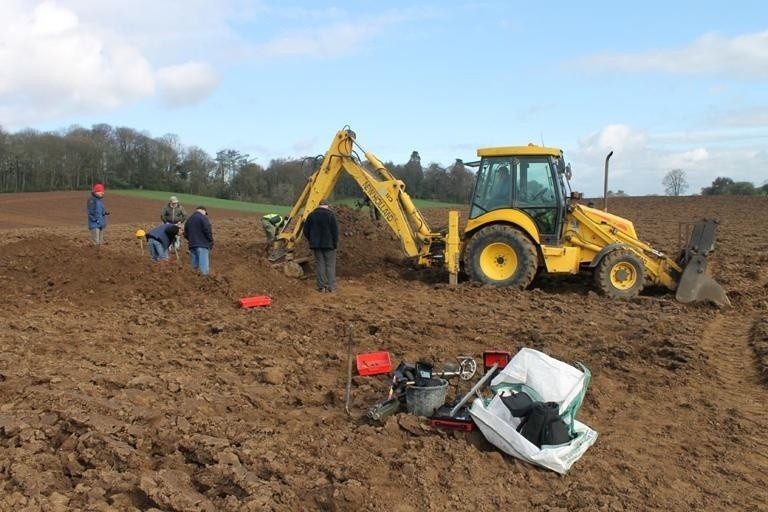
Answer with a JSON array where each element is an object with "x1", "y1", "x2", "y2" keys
[
  {"x1": 86, "y1": 183, "x2": 111, "y2": 245},
  {"x1": 354, "y1": 189, "x2": 381, "y2": 221},
  {"x1": 482, "y1": 166, "x2": 519, "y2": 212},
  {"x1": 160, "y1": 195, "x2": 189, "y2": 254},
  {"x1": 302, "y1": 198, "x2": 340, "y2": 294},
  {"x1": 183, "y1": 205, "x2": 215, "y2": 275},
  {"x1": 261, "y1": 212, "x2": 290, "y2": 255},
  {"x1": 146, "y1": 223, "x2": 185, "y2": 263}
]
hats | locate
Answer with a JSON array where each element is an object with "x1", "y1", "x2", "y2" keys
[
  {"x1": 94, "y1": 184, "x2": 105, "y2": 192},
  {"x1": 136, "y1": 230, "x2": 145, "y2": 238},
  {"x1": 196, "y1": 206, "x2": 208, "y2": 215},
  {"x1": 169, "y1": 196, "x2": 179, "y2": 204},
  {"x1": 319, "y1": 199, "x2": 329, "y2": 205}
]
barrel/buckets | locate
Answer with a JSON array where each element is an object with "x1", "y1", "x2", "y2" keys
[{"x1": 406, "y1": 380, "x2": 448, "y2": 418}]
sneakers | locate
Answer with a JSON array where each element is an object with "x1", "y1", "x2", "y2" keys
[{"x1": 320, "y1": 287, "x2": 336, "y2": 293}]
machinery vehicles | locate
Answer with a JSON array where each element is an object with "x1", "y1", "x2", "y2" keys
[{"x1": 262, "y1": 124, "x2": 732, "y2": 309}]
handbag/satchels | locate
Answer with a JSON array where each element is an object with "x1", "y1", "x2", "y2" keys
[{"x1": 499, "y1": 390, "x2": 574, "y2": 449}]
